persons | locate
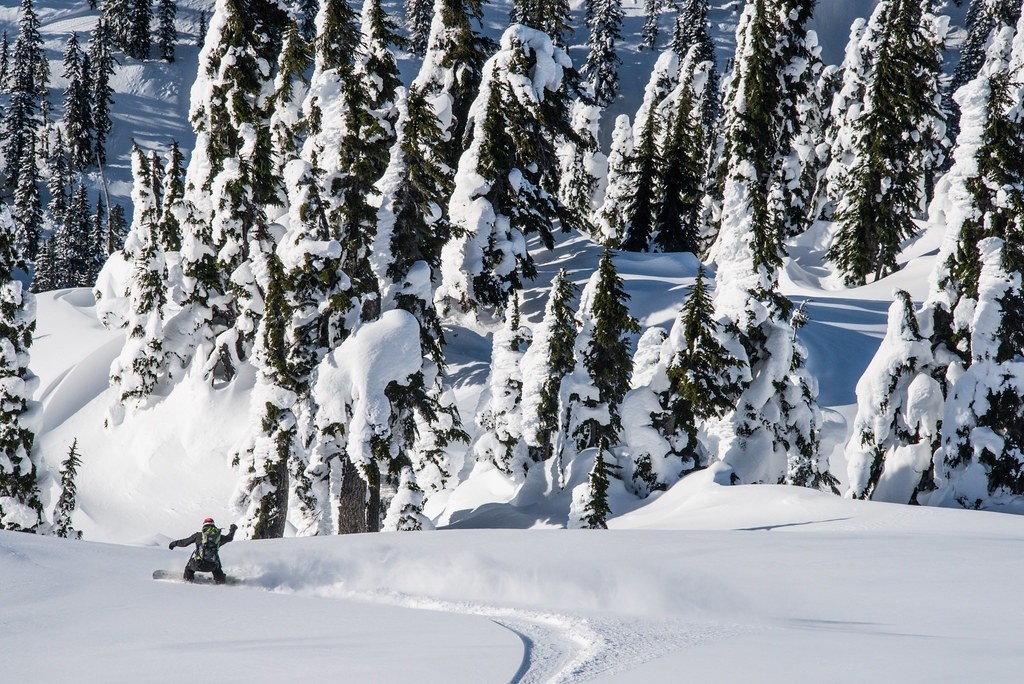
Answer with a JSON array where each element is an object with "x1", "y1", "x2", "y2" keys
[{"x1": 168, "y1": 517, "x2": 238, "y2": 584}]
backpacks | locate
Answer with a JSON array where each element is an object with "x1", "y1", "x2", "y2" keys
[{"x1": 200, "y1": 528, "x2": 221, "y2": 569}]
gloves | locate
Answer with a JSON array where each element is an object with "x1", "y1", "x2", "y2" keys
[
  {"x1": 169, "y1": 541, "x2": 175, "y2": 550},
  {"x1": 230, "y1": 524, "x2": 238, "y2": 530}
]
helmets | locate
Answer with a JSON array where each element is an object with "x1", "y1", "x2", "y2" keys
[{"x1": 203, "y1": 517, "x2": 214, "y2": 525}]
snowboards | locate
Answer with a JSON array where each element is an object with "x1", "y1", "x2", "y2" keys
[{"x1": 152, "y1": 570, "x2": 216, "y2": 585}]
